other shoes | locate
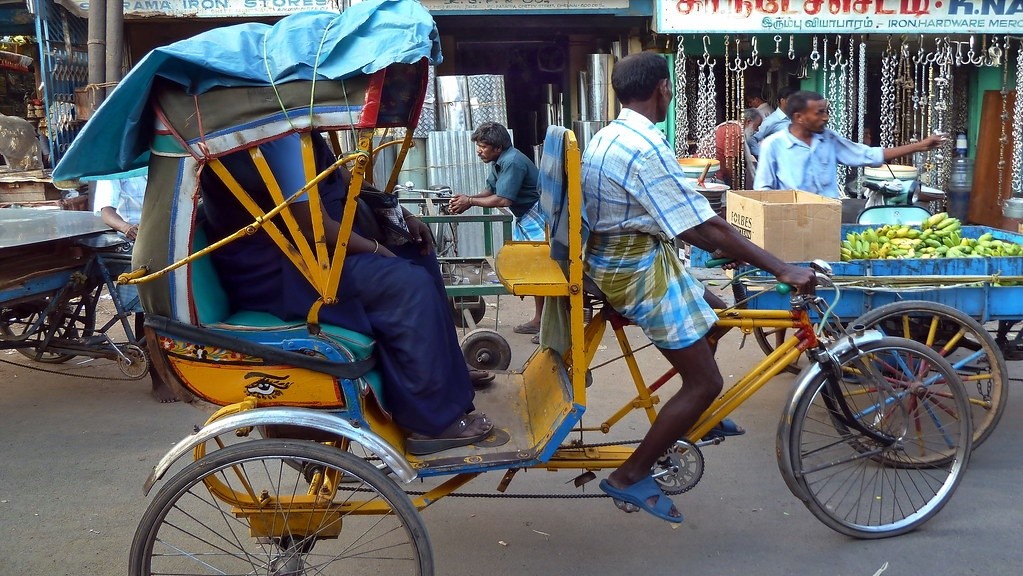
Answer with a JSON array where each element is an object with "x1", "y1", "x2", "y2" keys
[{"x1": 839, "y1": 369, "x2": 865, "y2": 385}]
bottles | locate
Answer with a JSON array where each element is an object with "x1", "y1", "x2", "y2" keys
[
  {"x1": 382, "y1": 195, "x2": 411, "y2": 244},
  {"x1": 949, "y1": 134, "x2": 973, "y2": 192},
  {"x1": 26, "y1": 98, "x2": 44, "y2": 130}
]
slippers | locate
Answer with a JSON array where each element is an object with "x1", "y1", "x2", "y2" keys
[
  {"x1": 514, "y1": 321, "x2": 540, "y2": 333},
  {"x1": 699, "y1": 418, "x2": 746, "y2": 442},
  {"x1": 403, "y1": 409, "x2": 494, "y2": 454},
  {"x1": 599, "y1": 476, "x2": 683, "y2": 522},
  {"x1": 465, "y1": 362, "x2": 495, "y2": 388},
  {"x1": 531, "y1": 332, "x2": 539, "y2": 344}
]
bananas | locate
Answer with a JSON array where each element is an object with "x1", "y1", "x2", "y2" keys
[{"x1": 841, "y1": 211, "x2": 1023, "y2": 286}]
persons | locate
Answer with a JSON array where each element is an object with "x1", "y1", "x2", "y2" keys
[
  {"x1": 199, "y1": 130, "x2": 495, "y2": 455},
  {"x1": 581, "y1": 52, "x2": 818, "y2": 523},
  {"x1": 753, "y1": 90, "x2": 948, "y2": 349},
  {"x1": 448, "y1": 121, "x2": 546, "y2": 344},
  {"x1": 94, "y1": 174, "x2": 145, "y2": 338},
  {"x1": 692, "y1": 85, "x2": 796, "y2": 189}
]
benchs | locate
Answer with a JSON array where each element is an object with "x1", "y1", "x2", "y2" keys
[{"x1": 190, "y1": 198, "x2": 378, "y2": 362}]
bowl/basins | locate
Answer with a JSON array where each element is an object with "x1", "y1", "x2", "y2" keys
[
  {"x1": 863, "y1": 163, "x2": 917, "y2": 182},
  {"x1": 676, "y1": 158, "x2": 731, "y2": 216}
]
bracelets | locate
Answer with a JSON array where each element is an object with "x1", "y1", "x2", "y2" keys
[
  {"x1": 370, "y1": 238, "x2": 378, "y2": 253},
  {"x1": 468, "y1": 197, "x2": 475, "y2": 206}
]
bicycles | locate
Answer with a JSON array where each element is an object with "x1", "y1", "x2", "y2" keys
[{"x1": 392, "y1": 181, "x2": 458, "y2": 284}]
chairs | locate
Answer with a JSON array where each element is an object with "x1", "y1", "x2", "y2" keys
[{"x1": 856, "y1": 205, "x2": 933, "y2": 225}]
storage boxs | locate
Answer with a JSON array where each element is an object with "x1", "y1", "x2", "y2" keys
[{"x1": 726, "y1": 189, "x2": 842, "y2": 263}]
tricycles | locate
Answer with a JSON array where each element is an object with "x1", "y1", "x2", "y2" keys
[
  {"x1": 127, "y1": 8, "x2": 975, "y2": 576},
  {"x1": 392, "y1": 179, "x2": 1023, "y2": 471},
  {"x1": 0, "y1": 207, "x2": 156, "y2": 381}
]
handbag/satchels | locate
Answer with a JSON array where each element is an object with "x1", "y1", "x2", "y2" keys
[{"x1": 358, "y1": 190, "x2": 417, "y2": 249}]
